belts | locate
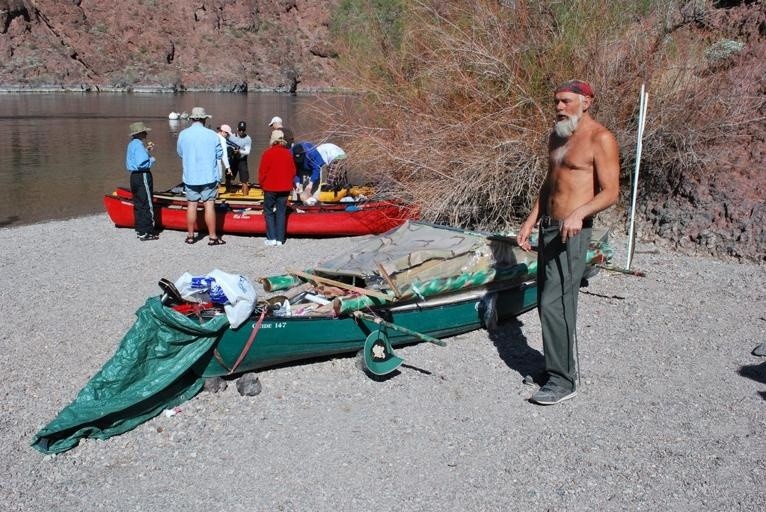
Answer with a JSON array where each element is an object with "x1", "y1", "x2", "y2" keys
[{"x1": 541, "y1": 216, "x2": 559, "y2": 227}]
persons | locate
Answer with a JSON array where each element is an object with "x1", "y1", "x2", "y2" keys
[
  {"x1": 269, "y1": 116, "x2": 294, "y2": 149},
  {"x1": 517, "y1": 78, "x2": 619, "y2": 404},
  {"x1": 177, "y1": 107, "x2": 226, "y2": 246},
  {"x1": 290, "y1": 142, "x2": 326, "y2": 202},
  {"x1": 125, "y1": 121, "x2": 159, "y2": 240},
  {"x1": 258, "y1": 130, "x2": 297, "y2": 245},
  {"x1": 316, "y1": 143, "x2": 348, "y2": 191},
  {"x1": 227, "y1": 121, "x2": 252, "y2": 195},
  {"x1": 217, "y1": 125, "x2": 233, "y2": 198}
]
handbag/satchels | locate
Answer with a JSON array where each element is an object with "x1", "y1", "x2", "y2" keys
[{"x1": 207, "y1": 270, "x2": 257, "y2": 330}]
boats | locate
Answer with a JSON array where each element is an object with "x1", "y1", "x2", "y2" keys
[
  {"x1": 101, "y1": 177, "x2": 419, "y2": 240},
  {"x1": 152, "y1": 228, "x2": 617, "y2": 380}
]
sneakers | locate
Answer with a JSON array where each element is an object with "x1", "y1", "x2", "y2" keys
[
  {"x1": 276, "y1": 241, "x2": 282, "y2": 246},
  {"x1": 523, "y1": 370, "x2": 577, "y2": 386},
  {"x1": 530, "y1": 380, "x2": 578, "y2": 404},
  {"x1": 264, "y1": 240, "x2": 276, "y2": 246},
  {"x1": 136, "y1": 231, "x2": 158, "y2": 240}
]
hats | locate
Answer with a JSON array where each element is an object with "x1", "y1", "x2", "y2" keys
[
  {"x1": 270, "y1": 130, "x2": 284, "y2": 145},
  {"x1": 294, "y1": 145, "x2": 305, "y2": 164},
  {"x1": 269, "y1": 117, "x2": 282, "y2": 127},
  {"x1": 221, "y1": 124, "x2": 232, "y2": 136},
  {"x1": 189, "y1": 108, "x2": 208, "y2": 119},
  {"x1": 129, "y1": 121, "x2": 152, "y2": 136},
  {"x1": 364, "y1": 330, "x2": 406, "y2": 375},
  {"x1": 238, "y1": 121, "x2": 245, "y2": 129}
]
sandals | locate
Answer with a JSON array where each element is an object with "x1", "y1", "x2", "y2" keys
[
  {"x1": 185, "y1": 236, "x2": 195, "y2": 244},
  {"x1": 208, "y1": 238, "x2": 226, "y2": 245}
]
